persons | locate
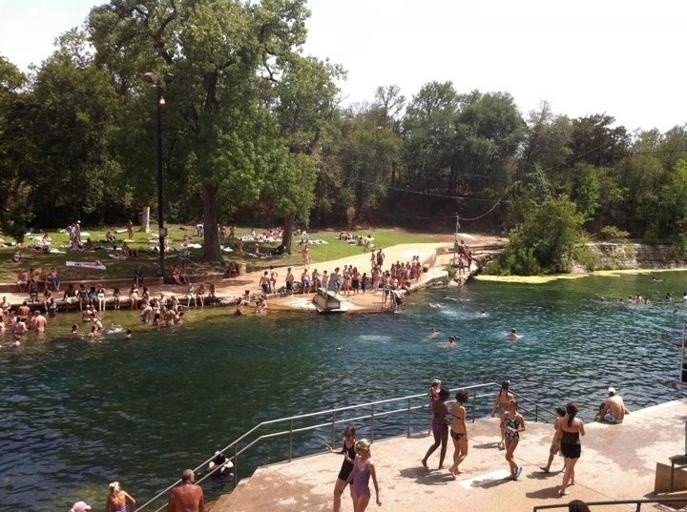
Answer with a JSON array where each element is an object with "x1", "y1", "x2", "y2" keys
[
  {"x1": 480, "y1": 309, "x2": 488, "y2": 317},
  {"x1": 168, "y1": 469, "x2": 204, "y2": 512},
  {"x1": 1, "y1": 219, "x2": 422, "y2": 348},
  {"x1": 431, "y1": 327, "x2": 455, "y2": 347},
  {"x1": 594, "y1": 387, "x2": 630, "y2": 424},
  {"x1": 71, "y1": 501, "x2": 91, "y2": 512},
  {"x1": 208, "y1": 452, "x2": 234, "y2": 477},
  {"x1": 422, "y1": 378, "x2": 469, "y2": 478},
  {"x1": 491, "y1": 380, "x2": 527, "y2": 478},
  {"x1": 540, "y1": 401, "x2": 585, "y2": 494},
  {"x1": 569, "y1": 500, "x2": 591, "y2": 512},
  {"x1": 326, "y1": 426, "x2": 382, "y2": 512},
  {"x1": 455, "y1": 238, "x2": 472, "y2": 276},
  {"x1": 106, "y1": 482, "x2": 135, "y2": 512},
  {"x1": 505, "y1": 327, "x2": 525, "y2": 340},
  {"x1": 600, "y1": 276, "x2": 687, "y2": 305}
]
